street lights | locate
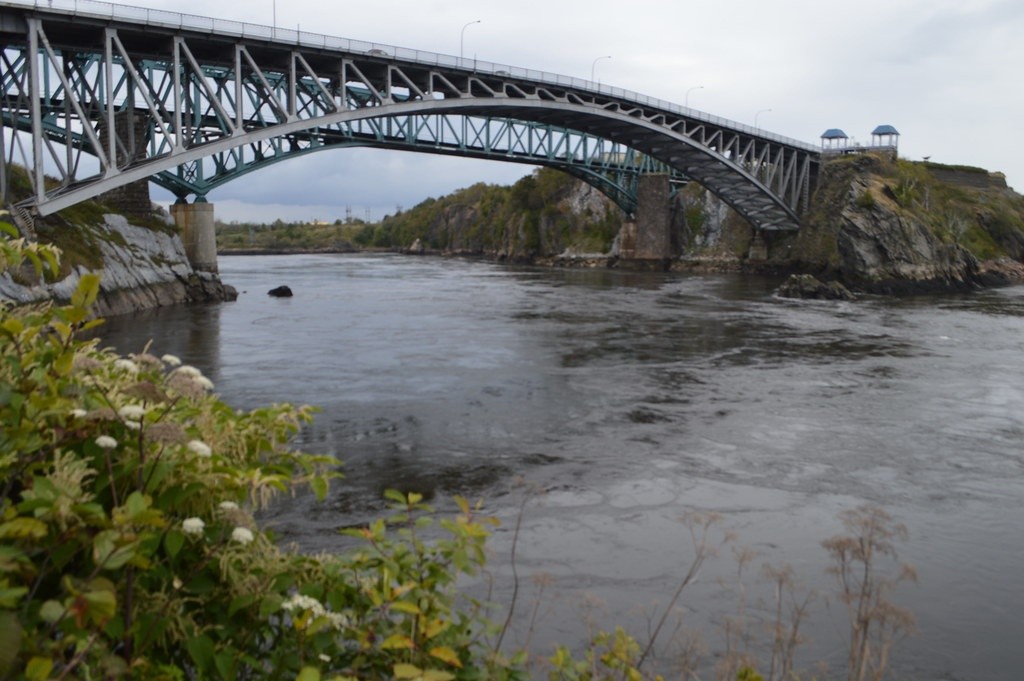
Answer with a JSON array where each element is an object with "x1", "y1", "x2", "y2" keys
[
  {"x1": 591, "y1": 56, "x2": 612, "y2": 82},
  {"x1": 460, "y1": 20, "x2": 480, "y2": 60},
  {"x1": 755, "y1": 108, "x2": 773, "y2": 128},
  {"x1": 686, "y1": 85, "x2": 705, "y2": 106}
]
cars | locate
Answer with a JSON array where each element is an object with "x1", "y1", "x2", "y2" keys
[
  {"x1": 496, "y1": 71, "x2": 511, "y2": 77},
  {"x1": 368, "y1": 49, "x2": 388, "y2": 55}
]
